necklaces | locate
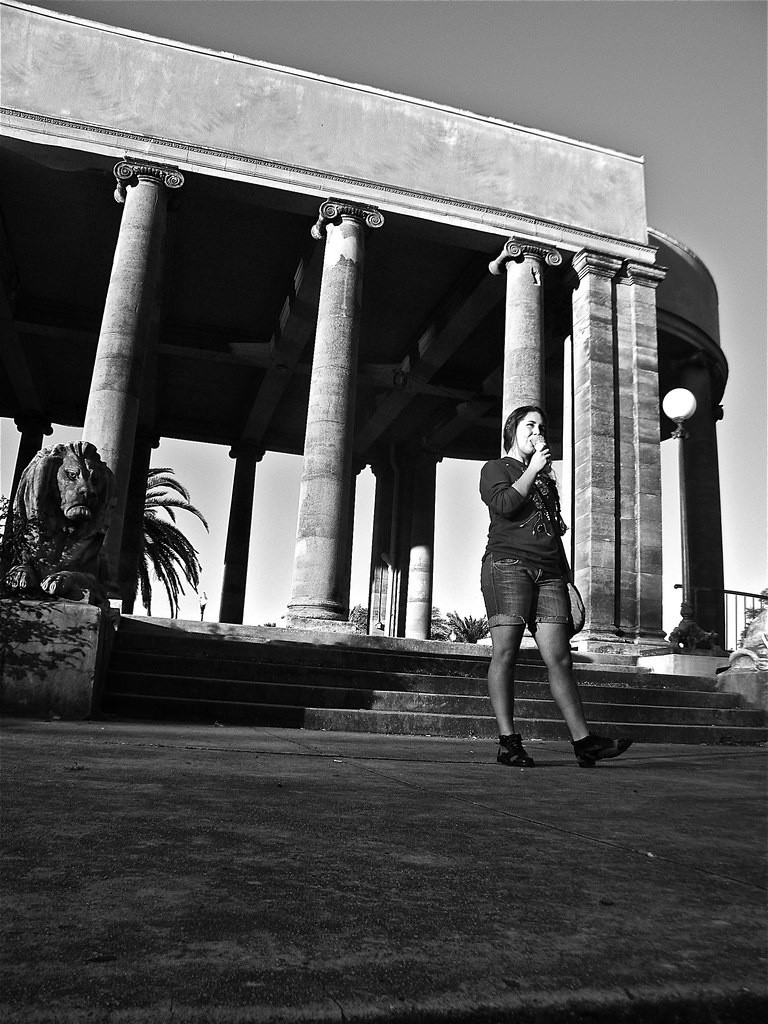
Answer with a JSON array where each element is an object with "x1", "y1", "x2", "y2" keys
[{"x1": 533, "y1": 481, "x2": 560, "y2": 524}]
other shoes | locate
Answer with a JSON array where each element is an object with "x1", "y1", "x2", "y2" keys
[
  {"x1": 571, "y1": 735, "x2": 633, "y2": 768},
  {"x1": 497, "y1": 734, "x2": 536, "y2": 767}
]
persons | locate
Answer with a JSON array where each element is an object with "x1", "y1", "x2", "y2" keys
[{"x1": 481, "y1": 405, "x2": 635, "y2": 768}]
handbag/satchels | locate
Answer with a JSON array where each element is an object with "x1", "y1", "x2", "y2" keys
[{"x1": 568, "y1": 583, "x2": 585, "y2": 638}]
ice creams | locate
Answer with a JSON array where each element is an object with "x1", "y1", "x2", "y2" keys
[{"x1": 530, "y1": 435, "x2": 545, "y2": 446}]
